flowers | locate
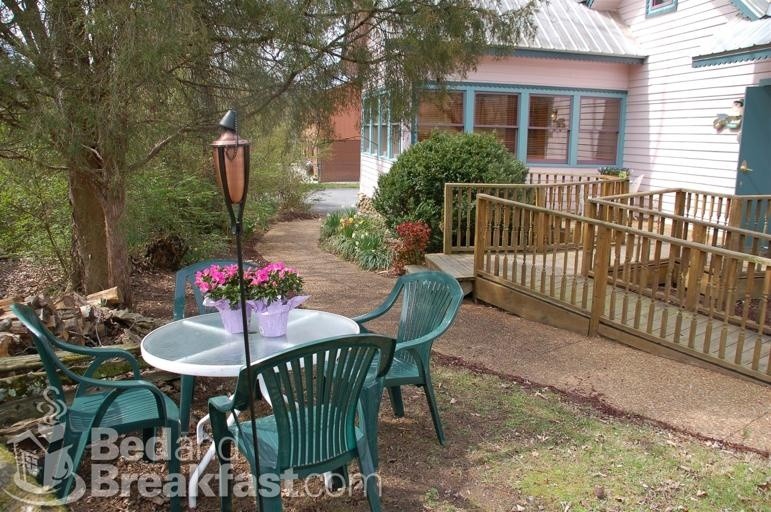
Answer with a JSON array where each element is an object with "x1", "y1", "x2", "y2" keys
[
  {"x1": 247, "y1": 261, "x2": 300, "y2": 303},
  {"x1": 192, "y1": 264, "x2": 248, "y2": 305}
]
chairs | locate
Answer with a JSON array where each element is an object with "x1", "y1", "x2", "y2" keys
[
  {"x1": 173, "y1": 259, "x2": 265, "y2": 435},
  {"x1": 207, "y1": 332, "x2": 384, "y2": 511},
  {"x1": 8, "y1": 300, "x2": 182, "y2": 512},
  {"x1": 323, "y1": 270, "x2": 463, "y2": 447}
]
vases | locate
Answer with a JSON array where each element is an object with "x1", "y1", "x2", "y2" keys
[
  {"x1": 255, "y1": 297, "x2": 307, "y2": 338},
  {"x1": 202, "y1": 302, "x2": 251, "y2": 335}
]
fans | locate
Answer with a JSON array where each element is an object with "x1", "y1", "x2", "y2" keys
[{"x1": 140, "y1": 308, "x2": 362, "y2": 508}]
